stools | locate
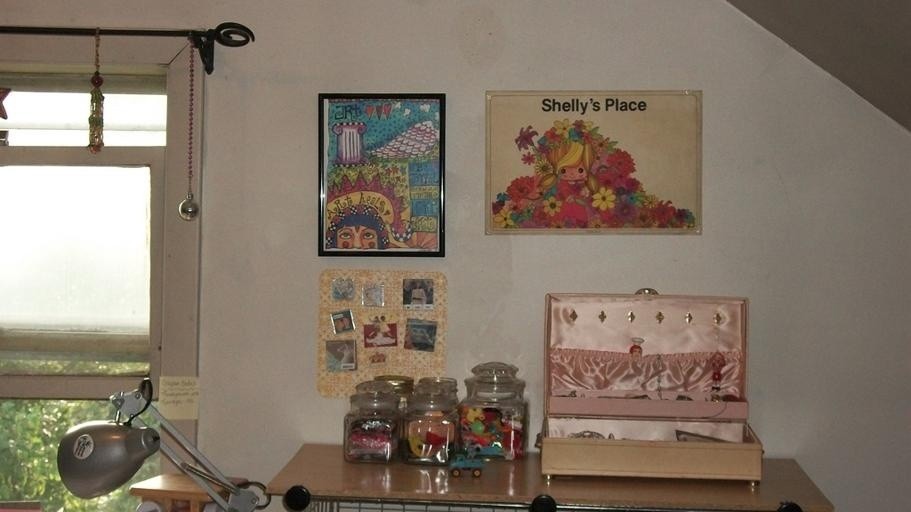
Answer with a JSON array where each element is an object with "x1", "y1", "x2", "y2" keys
[{"x1": 130, "y1": 473, "x2": 248, "y2": 512}]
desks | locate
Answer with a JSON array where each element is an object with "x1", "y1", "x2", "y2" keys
[{"x1": 263, "y1": 443, "x2": 835, "y2": 512}]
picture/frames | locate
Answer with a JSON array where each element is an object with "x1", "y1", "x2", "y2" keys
[
  {"x1": 318, "y1": 93, "x2": 446, "y2": 257},
  {"x1": 485, "y1": 89, "x2": 703, "y2": 236}
]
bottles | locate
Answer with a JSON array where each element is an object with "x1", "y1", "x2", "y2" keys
[{"x1": 343, "y1": 362, "x2": 529, "y2": 462}]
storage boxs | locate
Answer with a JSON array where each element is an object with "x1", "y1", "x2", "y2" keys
[{"x1": 541, "y1": 293, "x2": 763, "y2": 488}]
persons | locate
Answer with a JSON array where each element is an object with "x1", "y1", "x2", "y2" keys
[
  {"x1": 326, "y1": 170, "x2": 415, "y2": 249},
  {"x1": 404, "y1": 279, "x2": 415, "y2": 304},
  {"x1": 629, "y1": 336, "x2": 646, "y2": 356},
  {"x1": 336, "y1": 320, "x2": 345, "y2": 333},
  {"x1": 380, "y1": 315, "x2": 395, "y2": 338},
  {"x1": 424, "y1": 279, "x2": 434, "y2": 304},
  {"x1": 411, "y1": 279, "x2": 428, "y2": 304},
  {"x1": 367, "y1": 315, "x2": 381, "y2": 338},
  {"x1": 539, "y1": 141, "x2": 615, "y2": 225},
  {"x1": 707, "y1": 350, "x2": 729, "y2": 389},
  {"x1": 343, "y1": 317, "x2": 353, "y2": 330},
  {"x1": 337, "y1": 339, "x2": 356, "y2": 364}
]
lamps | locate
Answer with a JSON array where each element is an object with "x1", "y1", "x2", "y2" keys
[{"x1": 57, "y1": 377, "x2": 271, "y2": 512}]
